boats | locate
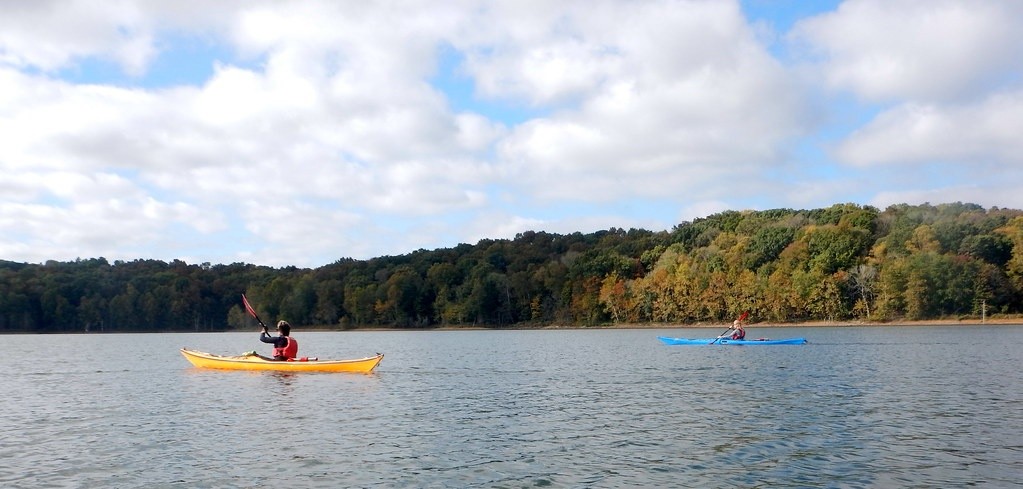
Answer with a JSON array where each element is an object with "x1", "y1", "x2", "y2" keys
[
  {"x1": 657, "y1": 335, "x2": 808, "y2": 345},
  {"x1": 180, "y1": 347, "x2": 386, "y2": 374}
]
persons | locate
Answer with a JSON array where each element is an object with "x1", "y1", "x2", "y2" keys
[
  {"x1": 717, "y1": 320, "x2": 745, "y2": 340},
  {"x1": 260, "y1": 320, "x2": 298, "y2": 361}
]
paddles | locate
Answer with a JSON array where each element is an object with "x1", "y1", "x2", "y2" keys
[
  {"x1": 242, "y1": 293, "x2": 270, "y2": 336},
  {"x1": 709, "y1": 312, "x2": 748, "y2": 344}
]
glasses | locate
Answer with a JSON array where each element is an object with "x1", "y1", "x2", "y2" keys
[{"x1": 276, "y1": 328, "x2": 281, "y2": 331}]
